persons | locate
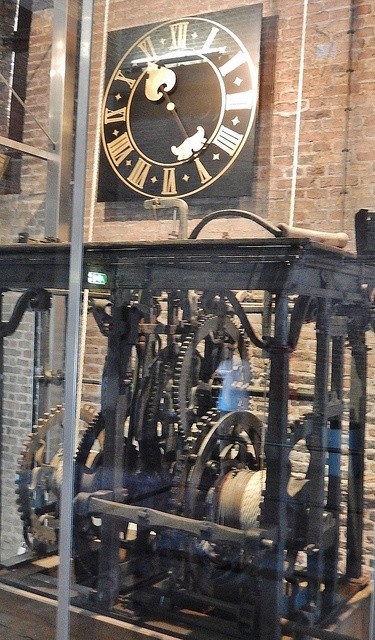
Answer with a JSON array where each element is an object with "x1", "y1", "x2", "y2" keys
[{"x1": 210, "y1": 342, "x2": 251, "y2": 488}]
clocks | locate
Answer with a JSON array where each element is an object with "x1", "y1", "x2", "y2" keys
[{"x1": 96, "y1": 2, "x2": 263, "y2": 203}]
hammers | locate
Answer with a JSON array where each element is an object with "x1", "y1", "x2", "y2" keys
[{"x1": 17, "y1": 232, "x2": 48, "y2": 244}]
